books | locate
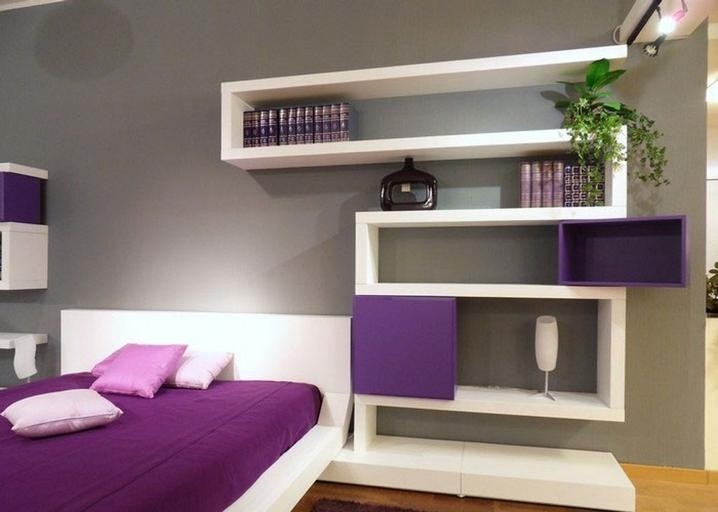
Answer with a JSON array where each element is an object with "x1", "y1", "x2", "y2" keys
[
  {"x1": 243, "y1": 102, "x2": 358, "y2": 146},
  {"x1": 519, "y1": 160, "x2": 603, "y2": 207}
]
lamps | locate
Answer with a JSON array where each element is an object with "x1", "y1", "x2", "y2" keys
[
  {"x1": 659, "y1": 0, "x2": 689, "y2": 35},
  {"x1": 642, "y1": 6, "x2": 666, "y2": 58}
]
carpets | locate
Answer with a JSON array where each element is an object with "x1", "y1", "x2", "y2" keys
[{"x1": 311, "y1": 496, "x2": 414, "y2": 512}]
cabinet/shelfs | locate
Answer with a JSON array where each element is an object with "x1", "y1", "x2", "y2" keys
[
  {"x1": 220, "y1": 44, "x2": 688, "y2": 511},
  {"x1": 0, "y1": 162, "x2": 51, "y2": 353}
]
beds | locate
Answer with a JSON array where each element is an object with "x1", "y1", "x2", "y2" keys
[{"x1": 1, "y1": 309, "x2": 354, "y2": 511}]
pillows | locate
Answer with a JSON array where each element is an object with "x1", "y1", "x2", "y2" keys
[
  {"x1": 88, "y1": 344, "x2": 188, "y2": 399},
  {"x1": 162, "y1": 353, "x2": 232, "y2": 390},
  {"x1": 90, "y1": 344, "x2": 128, "y2": 377},
  {"x1": 1, "y1": 388, "x2": 125, "y2": 439}
]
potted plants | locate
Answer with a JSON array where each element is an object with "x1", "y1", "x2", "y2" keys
[{"x1": 552, "y1": 58, "x2": 671, "y2": 204}]
